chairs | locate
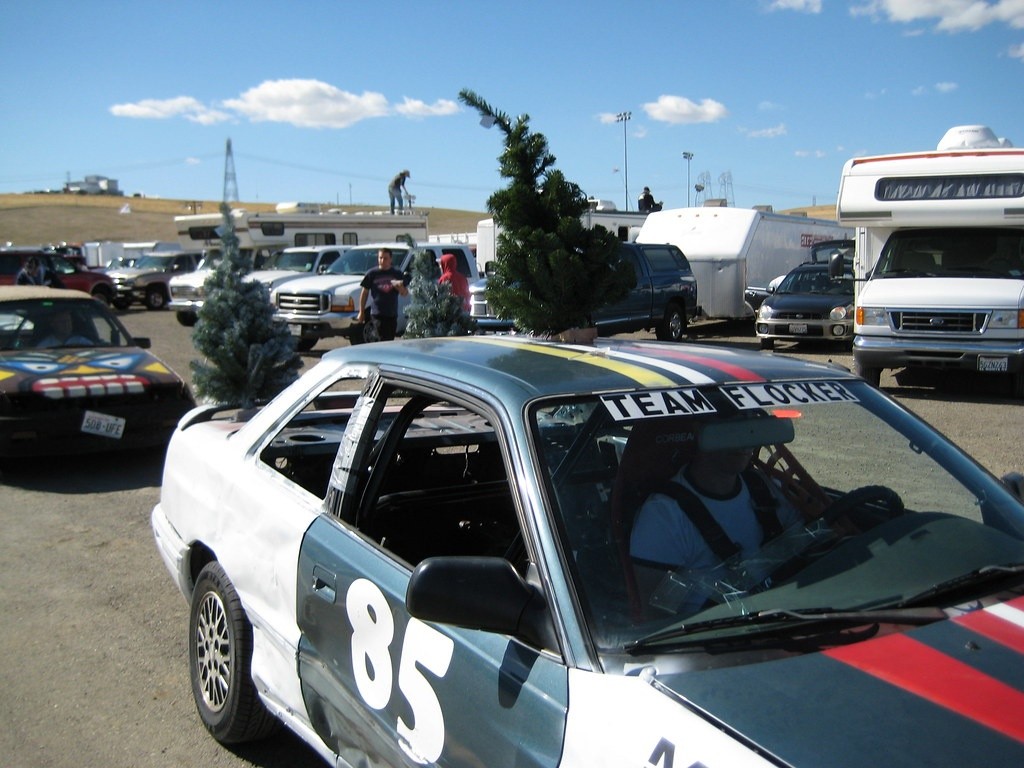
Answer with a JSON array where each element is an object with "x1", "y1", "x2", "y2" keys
[
  {"x1": 609, "y1": 413, "x2": 708, "y2": 624},
  {"x1": 28, "y1": 312, "x2": 95, "y2": 348},
  {"x1": 894, "y1": 252, "x2": 941, "y2": 274},
  {"x1": 798, "y1": 278, "x2": 812, "y2": 291}
]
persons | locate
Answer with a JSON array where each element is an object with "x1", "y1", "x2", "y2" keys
[
  {"x1": 357, "y1": 248, "x2": 407, "y2": 342},
  {"x1": 389, "y1": 169, "x2": 411, "y2": 215},
  {"x1": 424, "y1": 249, "x2": 472, "y2": 314},
  {"x1": 16, "y1": 257, "x2": 52, "y2": 286},
  {"x1": 638, "y1": 186, "x2": 662, "y2": 213},
  {"x1": 630, "y1": 411, "x2": 820, "y2": 615},
  {"x1": 36, "y1": 309, "x2": 93, "y2": 348}
]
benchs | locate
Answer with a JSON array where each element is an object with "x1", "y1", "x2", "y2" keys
[{"x1": 364, "y1": 426, "x2": 617, "y2": 536}]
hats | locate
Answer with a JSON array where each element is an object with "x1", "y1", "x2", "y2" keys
[
  {"x1": 436, "y1": 257, "x2": 441, "y2": 262},
  {"x1": 404, "y1": 169, "x2": 410, "y2": 178}
]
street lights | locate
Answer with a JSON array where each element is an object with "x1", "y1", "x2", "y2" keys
[
  {"x1": 682, "y1": 152, "x2": 694, "y2": 208},
  {"x1": 615, "y1": 111, "x2": 633, "y2": 211},
  {"x1": 694, "y1": 184, "x2": 705, "y2": 207}
]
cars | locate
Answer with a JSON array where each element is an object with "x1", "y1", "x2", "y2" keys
[
  {"x1": 88, "y1": 256, "x2": 136, "y2": 276},
  {"x1": 742, "y1": 237, "x2": 861, "y2": 350},
  {"x1": 0, "y1": 245, "x2": 118, "y2": 313}
]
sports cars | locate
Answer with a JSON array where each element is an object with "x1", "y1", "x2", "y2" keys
[
  {"x1": 0, "y1": 285, "x2": 195, "y2": 465},
  {"x1": 150, "y1": 333, "x2": 1024, "y2": 767}
]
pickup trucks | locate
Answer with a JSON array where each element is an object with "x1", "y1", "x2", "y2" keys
[{"x1": 469, "y1": 243, "x2": 700, "y2": 344}]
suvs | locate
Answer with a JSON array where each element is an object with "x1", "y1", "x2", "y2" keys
[
  {"x1": 236, "y1": 243, "x2": 357, "y2": 293},
  {"x1": 101, "y1": 251, "x2": 205, "y2": 309},
  {"x1": 268, "y1": 244, "x2": 481, "y2": 355}
]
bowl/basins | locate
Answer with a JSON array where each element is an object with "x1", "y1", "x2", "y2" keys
[{"x1": 390, "y1": 280, "x2": 403, "y2": 287}]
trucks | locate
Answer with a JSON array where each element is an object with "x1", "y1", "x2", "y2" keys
[
  {"x1": 164, "y1": 202, "x2": 429, "y2": 324},
  {"x1": 834, "y1": 124, "x2": 1024, "y2": 402}
]
